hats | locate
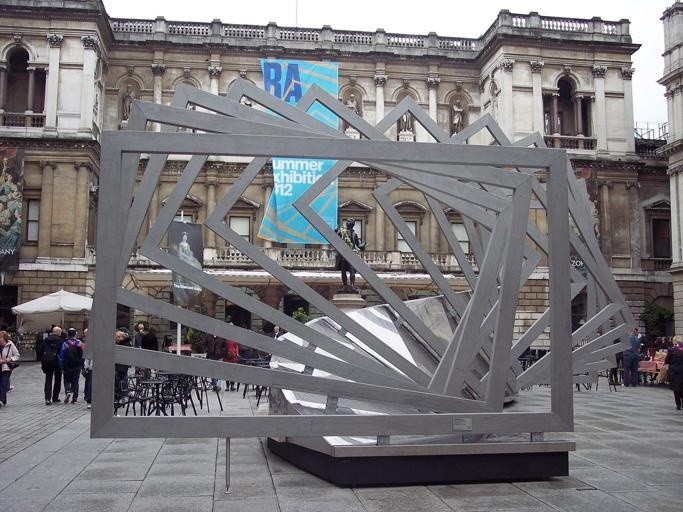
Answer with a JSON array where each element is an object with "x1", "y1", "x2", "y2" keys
[{"x1": 68, "y1": 328, "x2": 76, "y2": 336}]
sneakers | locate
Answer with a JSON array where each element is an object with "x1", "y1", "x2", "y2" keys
[
  {"x1": 83, "y1": 395, "x2": 91, "y2": 409},
  {"x1": 226, "y1": 386, "x2": 235, "y2": 391},
  {"x1": 45, "y1": 393, "x2": 80, "y2": 405},
  {"x1": 610, "y1": 380, "x2": 638, "y2": 387},
  {"x1": 7, "y1": 386, "x2": 14, "y2": 393}
]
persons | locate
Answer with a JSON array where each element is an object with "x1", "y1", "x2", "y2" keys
[
  {"x1": 664, "y1": 334, "x2": 683, "y2": 411},
  {"x1": 0, "y1": 328, "x2": 20, "y2": 408},
  {"x1": 0, "y1": 155, "x2": 22, "y2": 239},
  {"x1": 5, "y1": 332, "x2": 14, "y2": 392},
  {"x1": 608, "y1": 338, "x2": 623, "y2": 386},
  {"x1": 450, "y1": 99, "x2": 465, "y2": 137},
  {"x1": 620, "y1": 330, "x2": 642, "y2": 387},
  {"x1": 333, "y1": 216, "x2": 367, "y2": 296},
  {"x1": 172, "y1": 230, "x2": 204, "y2": 291},
  {"x1": 398, "y1": 109, "x2": 413, "y2": 134},
  {"x1": 36, "y1": 319, "x2": 281, "y2": 410},
  {"x1": 345, "y1": 93, "x2": 362, "y2": 131},
  {"x1": 633, "y1": 327, "x2": 653, "y2": 386}
]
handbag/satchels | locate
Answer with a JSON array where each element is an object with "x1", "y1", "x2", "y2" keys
[
  {"x1": 7, "y1": 361, "x2": 19, "y2": 369},
  {"x1": 82, "y1": 368, "x2": 90, "y2": 378}
]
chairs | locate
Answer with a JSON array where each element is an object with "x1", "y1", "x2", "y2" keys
[
  {"x1": 112, "y1": 356, "x2": 270, "y2": 416},
  {"x1": 596, "y1": 366, "x2": 618, "y2": 392}
]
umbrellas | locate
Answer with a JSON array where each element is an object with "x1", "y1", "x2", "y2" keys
[{"x1": 9, "y1": 289, "x2": 93, "y2": 328}]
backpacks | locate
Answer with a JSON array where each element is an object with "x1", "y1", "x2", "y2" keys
[
  {"x1": 42, "y1": 341, "x2": 62, "y2": 366},
  {"x1": 65, "y1": 340, "x2": 81, "y2": 368}
]
